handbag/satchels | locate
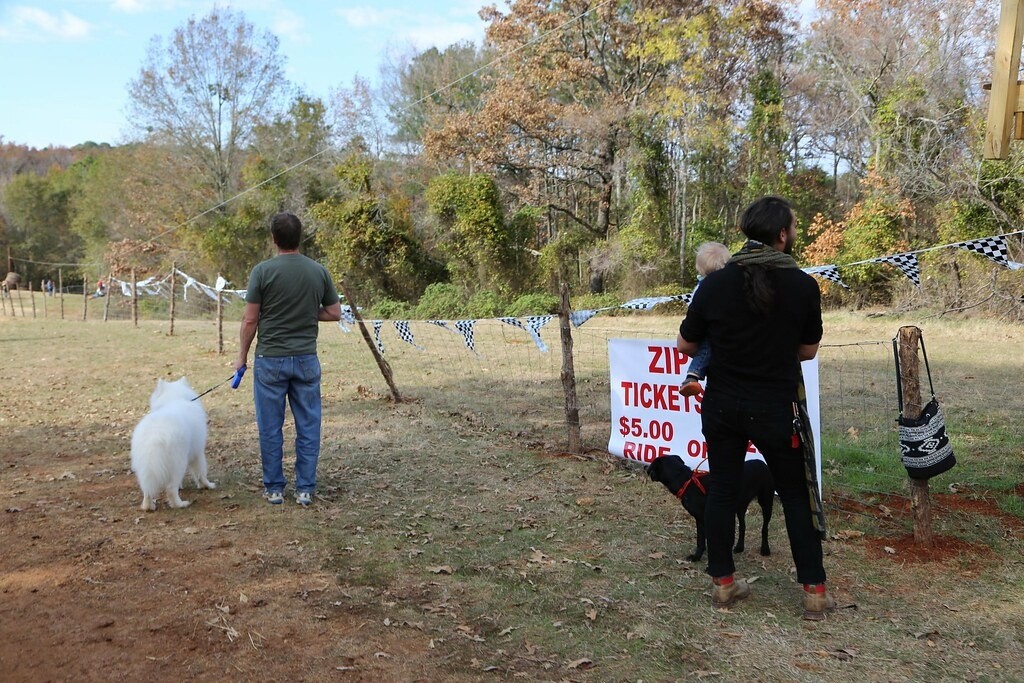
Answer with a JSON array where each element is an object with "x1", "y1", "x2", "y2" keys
[{"x1": 892, "y1": 326, "x2": 957, "y2": 482}]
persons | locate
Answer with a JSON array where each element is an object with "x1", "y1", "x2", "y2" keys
[
  {"x1": 2, "y1": 281, "x2": 10, "y2": 298},
  {"x1": 234, "y1": 213, "x2": 342, "y2": 505},
  {"x1": 88, "y1": 281, "x2": 107, "y2": 300},
  {"x1": 47, "y1": 279, "x2": 54, "y2": 296},
  {"x1": 679, "y1": 240, "x2": 732, "y2": 397},
  {"x1": 677, "y1": 196, "x2": 837, "y2": 620}
]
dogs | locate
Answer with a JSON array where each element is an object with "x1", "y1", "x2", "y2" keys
[
  {"x1": 130, "y1": 375, "x2": 217, "y2": 513},
  {"x1": 648, "y1": 455, "x2": 776, "y2": 577}
]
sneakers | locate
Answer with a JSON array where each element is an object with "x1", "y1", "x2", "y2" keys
[
  {"x1": 290, "y1": 489, "x2": 311, "y2": 505},
  {"x1": 262, "y1": 488, "x2": 283, "y2": 504}
]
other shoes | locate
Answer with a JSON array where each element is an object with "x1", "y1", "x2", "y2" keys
[
  {"x1": 801, "y1": 589, "x2": 834, "y2": 620},
  {"x1": 679, "y1": 381, "x2": 702, "y2": 398},
  {"x1": 710, "y1": 579, "x2": 750, "y2": 605}
]
